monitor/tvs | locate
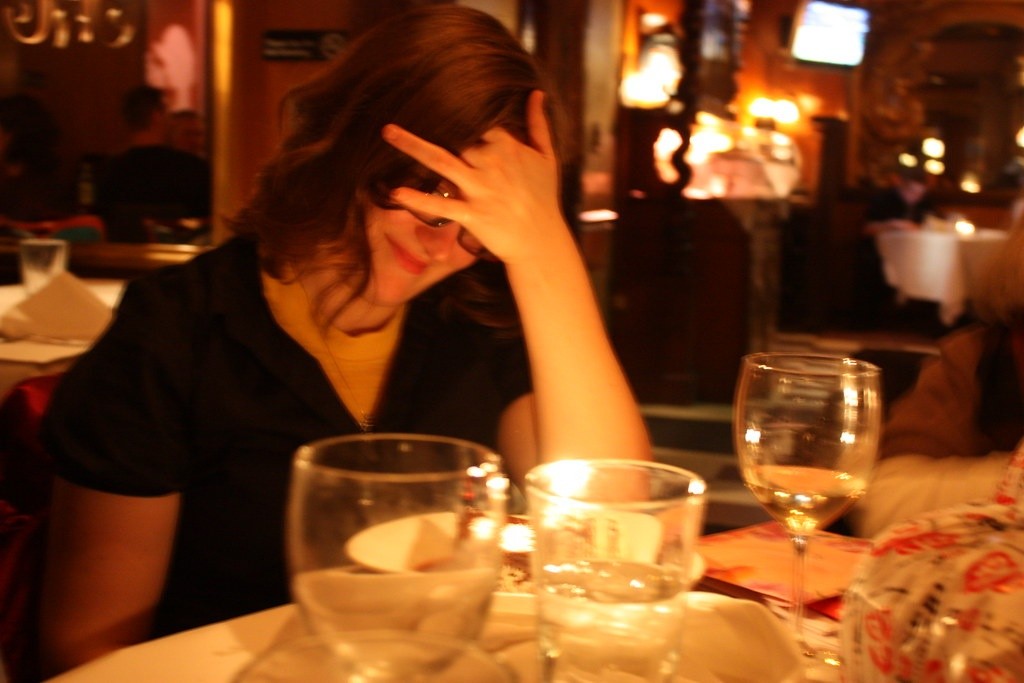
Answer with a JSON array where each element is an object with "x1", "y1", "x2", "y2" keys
[{"x1": 789, "y1": 0, "x2": 870, "y2": 66}]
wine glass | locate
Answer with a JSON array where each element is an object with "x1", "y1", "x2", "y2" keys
[{"x1": 732, "y1": 351, "x2": 885, "y2": 683}]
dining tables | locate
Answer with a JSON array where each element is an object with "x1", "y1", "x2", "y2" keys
[
  {"x1": 53, "y1": 604, "x2": 840, "y2": 683},
  {"x1": 0, "y1": 280, "x2": 125, "y2": 397},
  {"x1": 879, "y1": 226, "x2": 1012, "y2": 327}
]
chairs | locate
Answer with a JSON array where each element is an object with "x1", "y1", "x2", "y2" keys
[
  {"x1": 1, "y1": 215, "x2": 107, "y2": 241},
  {"x1": 145, "y1": 217, "x2": 210, "y2": 243}
]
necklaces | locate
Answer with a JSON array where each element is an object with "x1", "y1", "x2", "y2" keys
[{"x1": 278, "y1": 249, "x2": 406, "y2": 433}]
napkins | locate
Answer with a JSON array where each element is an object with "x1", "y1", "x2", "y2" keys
[
  {"x1": 476, "y1": 592, "x2": 808, "y2": 683},
  {"x1": 0, "y1": 267, "x2": 116, "y2": 346}
]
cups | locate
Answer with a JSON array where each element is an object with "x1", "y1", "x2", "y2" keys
[
  {"x1": 20, "y1": 239, "x2": 67, "y2": 300},
  {"x1": 523, "y1": 456, "x2": 712, "y2": 683},
  {"x1": 286, "y1": 434, "x2": 512, "y2": 683}
]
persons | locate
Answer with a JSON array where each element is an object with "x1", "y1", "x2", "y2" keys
[
  {"x1": 166, "y1": 108, "x2": 206, "y2": 160},
  {"x1": 840, "y1": 198, "x2": 1024, "y2": 538},
  {"x1": 856, "y1": 167, "x2": 965, "y2": 215},
  {"x1": 32, "y1": 3, "x2": 655, "y2": 678},
  {"x1": 91, "y1": 83, "x2": 211, "y2": 249},
  {"x1": 1, "y1": 95, "x2": 80, "y2": 221}
]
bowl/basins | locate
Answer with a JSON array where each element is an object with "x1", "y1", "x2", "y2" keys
[{"x1": 345, "y1": 511, "x2": 707, "y2": 642}]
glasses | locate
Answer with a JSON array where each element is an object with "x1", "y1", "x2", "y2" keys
[{"x1": 405, "y1": 180, "x2": 502, "y2": 264}]
